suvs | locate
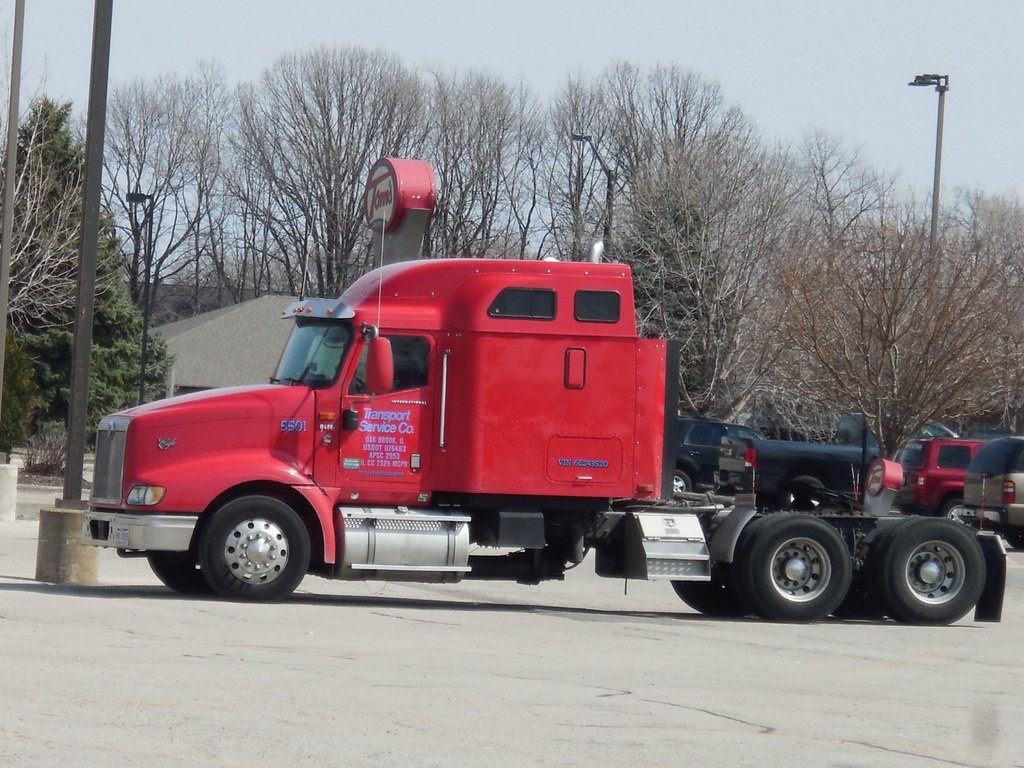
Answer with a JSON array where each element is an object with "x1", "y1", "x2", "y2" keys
[{"x1": 674, "y1": 411, "x2": 772, "y2": 499}]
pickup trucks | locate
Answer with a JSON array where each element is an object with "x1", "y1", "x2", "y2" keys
[{"x1": 706, "y1": 414, "x2": 964, "y2": 509}]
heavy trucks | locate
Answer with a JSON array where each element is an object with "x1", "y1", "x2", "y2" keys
[{"x1": 84, "y1": 255, "x2": 1011, "y2": 629}]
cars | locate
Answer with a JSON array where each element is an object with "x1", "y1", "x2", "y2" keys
[
  {"x1": 891, "y1": 437, "x2": 988, "y2": 529},
  {"x1": 961, "y1": 435, "x2": 1024, "y2": 551}
]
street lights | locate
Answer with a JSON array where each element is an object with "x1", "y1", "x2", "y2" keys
[
  {"x1": 569, "y1": 131, "x2": 617, "y2": 260},
  {"x1": 909, "y1": 71, "x2": 953, "y2": 286},
  {"x1": 126, "y1": 191, "x2": 155, "y2": 400}
]
